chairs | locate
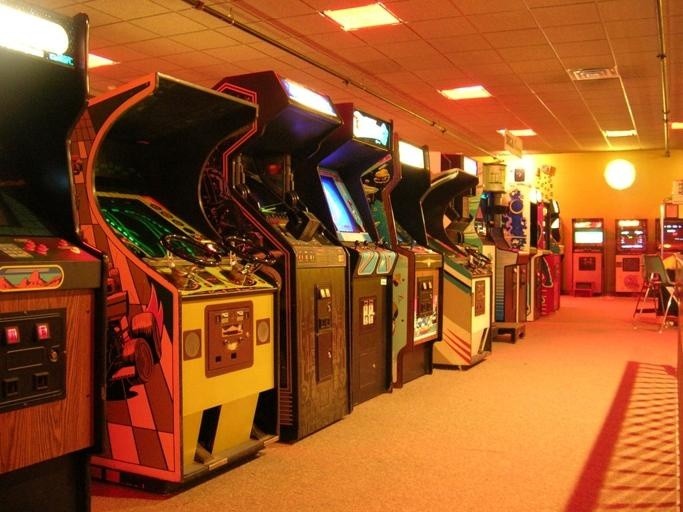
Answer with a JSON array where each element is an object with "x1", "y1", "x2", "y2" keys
[{"x1": 631, "y1": 252, "x2": 682, "y2": 334}]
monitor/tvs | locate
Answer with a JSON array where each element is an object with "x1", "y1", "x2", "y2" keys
[
  {"x1": 97, "y1": 191, "x2": 241, "y2": 265},
  {"x1": 574, "y1": 229, "x2": 605, "y2": 246},
  {"x1": 316, "y1": 166, "x2": 374, "y2": 242},
  {"x1": 0, "y1": 190, "x2": 55, "y2": 236},
  {"x1": 620, "y1": 230, "x2": 646, "y2": 249},
  {"x1": 664, "y1": 224, "x2": 683, "y2": 251}
]
